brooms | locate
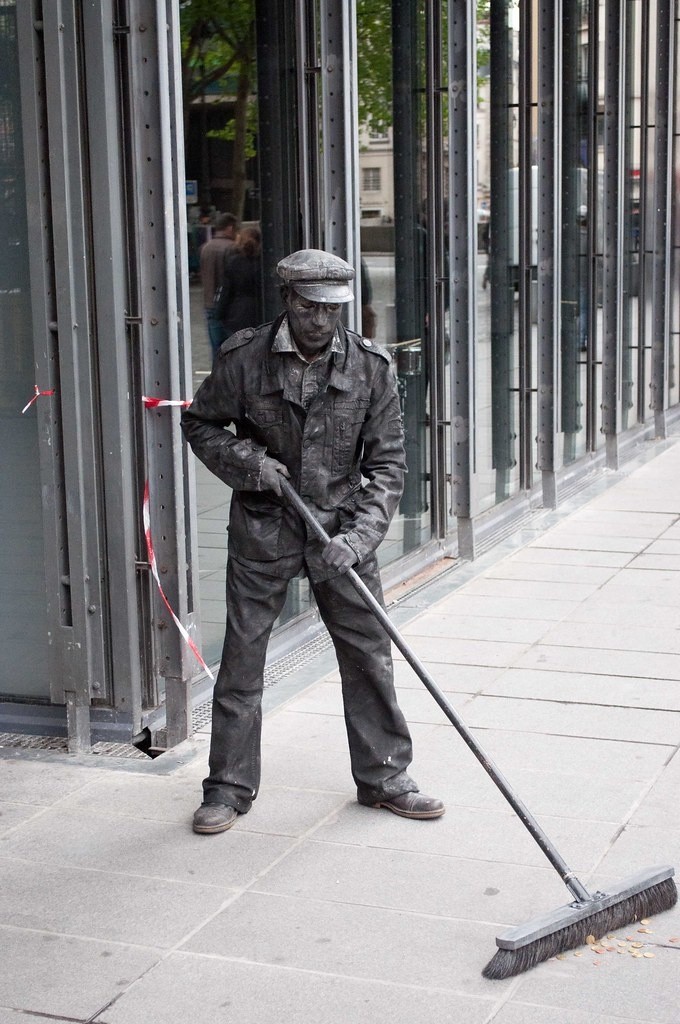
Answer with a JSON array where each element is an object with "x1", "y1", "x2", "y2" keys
[{"x1": 276, "y1": 470, "x2": 679, "y2": 982}]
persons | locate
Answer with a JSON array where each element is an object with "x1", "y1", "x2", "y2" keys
[
  {"x1": 215, "y1": 223, "x2": 271, "y2": 344},
  {"x1": 179, "y1": 248, "x2": 446, "y2": 835},
  {"x1": 200, "y1": 213, "x2": 257, "y2": 361}
]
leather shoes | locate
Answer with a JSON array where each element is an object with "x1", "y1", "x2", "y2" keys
[
  {"x1": 194, "y1": 803, "x2": 237, "y2": 833},
  {"x1": 358, "y1": 791, "x2": 445, "y2": 818}
]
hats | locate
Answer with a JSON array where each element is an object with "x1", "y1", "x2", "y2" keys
[{"x1": 277, "y1": 249, "x2": 355, "y2": 303}]
